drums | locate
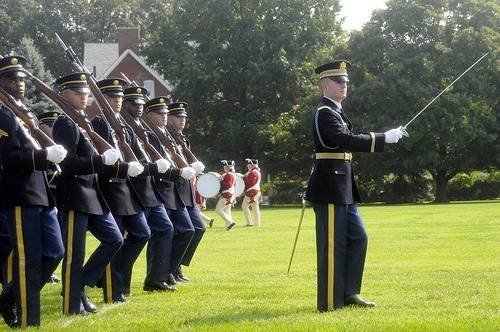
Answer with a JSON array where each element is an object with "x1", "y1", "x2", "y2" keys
[
  {"x1": 196, "y1": 171, "x2": 223, "y2": 198},
  {"x1": 235, "y1": 172, "x2": 245, "y2": 197}
]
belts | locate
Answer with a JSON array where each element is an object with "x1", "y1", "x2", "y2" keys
[{"x1": 315, "y1": 153, "x2": 353, "y2": 160}]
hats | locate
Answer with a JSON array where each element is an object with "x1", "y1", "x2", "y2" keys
[
  {"x1": 245, "y1": 158, "x2": 259, "y2": 164},
  {"x1": 37, "y1": 112, "x2": 60, "y2": 125},
  {"x1": 168, "y1": 102, "x2": 188, "y2": 117},
  {"x1": 96, "y1": 79, "x2": 126, "y2": 96},
  {"x1": 315, "y1": 60, "x2": 351, "y2": 82},
  {"x1": 0, "y1": 55, "x2": 28, "y2": 78},
  {"x1": 221, "y1": 160, "x2": 236, "y2": 166},
  {"x1": 144, "y1": 97, "x2": 170, "y2": 113},
  {"x1": 56, "y1": 73, "x2": 92, "y2": 93},
  {"x1": 122, "y1": 87, "x2": 147, "y2": 104}
]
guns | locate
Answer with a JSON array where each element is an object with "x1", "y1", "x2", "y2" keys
[
  {"x1": 121, "y1": 73, "x2": 197, "y2": 163},
  {"x1": 104, "y1": 75, "x2": 189, "y2": 168},
  {"x1": 55, "y1": 32, "x2": 139, "y2": 163},
  {"x1": 0, "y1": 54, "x2": 115, "y2": 154},
  {"x1": 84, "y1": 66, "x2": 163, "y2": 161},
  {"x1": 0, "y1": 85, "x2": 58, "y2": 165}
]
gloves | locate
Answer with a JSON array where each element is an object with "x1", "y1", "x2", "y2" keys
[
  {"x1": 191, "y1": 161, "x2": 205, "y2": 175},
  {"x1": 103, "y1": 149, "x2": 120, "y2": 166},
  {"x1": 384, "y1": 126, "x2": 403, "y2": 143},
  {"x1": 180, "y1": 167, "x2": 196, "y2": 180},
  {"x1": 155, "y1": 159, "x2": 171, "y2": 174},
  {"x1": 46, "y1": 145, "x2": 68, "y2": 163},
  {"x1": 127, "y1": 161, "x2": 144, "y2": 177}
]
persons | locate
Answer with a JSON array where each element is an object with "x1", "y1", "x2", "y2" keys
[
  {"x1": 90, "y1": 79, "x2": 151, "y2": 301},
  {"x1": 37, "y1": 112, "x2": 65, "y2": 283},
  {"x1": 53, "y1": 72, "x2": 124, "y2": 316},
  {"x1": 144, "y1": 99, "x2": 206, "y2": 285},
  {"x1": 120, "y1": 86, "x2": 178, "y2": 292},
  {"x1": 242, "y1": 159, "x2": 262, "y2": 227},
  {"x1": 196, "y1": 173, "x2": 214, "y2": 227},
  {"x1": 216, "y1": 160, "x2": 236, "y2": 230},
  {"x1": 304, "y1": 60, "x2": 405, "y2": 312},
  {"x1": 0, "y1": 56, "x2": 68, "y2": 328}
]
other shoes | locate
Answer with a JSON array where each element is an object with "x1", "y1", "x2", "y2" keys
[
  {"x1": 143, "y1": 276, "x2": 178, "y2": 292},
  {"x1": 61, "y1": 289, "x2": 98, "y2": 317},
  {"x1": 344, "y1": 294, "x2": 376, "y2": 308},
  {"x1": 170, "y1": 267, "x2": 190, "y2": 282},
  {"x1": 46, "y1": 274, "x2": 61, "y2": 283},
  {"x1": 227, "y1": 222, "x2": 235, "y2": 230},
  {"x1": 104, "y1": 284, "x2": 126, "y2": 303},
  {"x1": 209, "y1": 219, "x2": 214, "y2": 228},
  {"x1": 166, "y1": 273, "x2": 177, "y2": 284},
  {"x1": 0, "y1": 294, "x2": 18, "y2": 329}
]
